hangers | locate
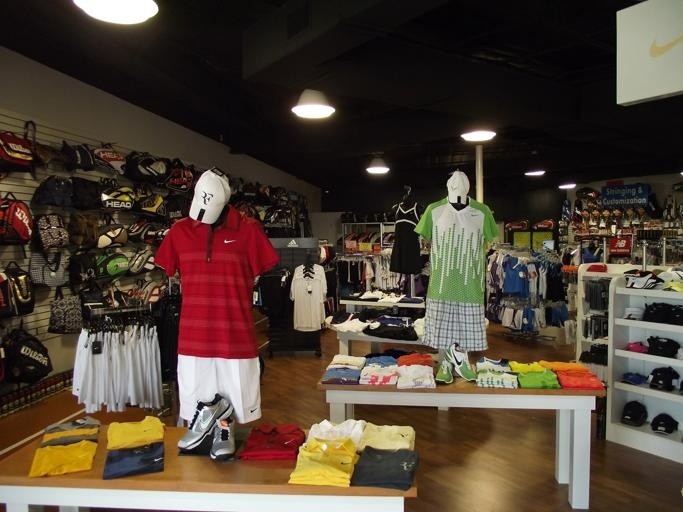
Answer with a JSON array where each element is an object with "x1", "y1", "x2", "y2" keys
[{"x1": 292, "y1": 251, "x2": 323, "y2": 282}]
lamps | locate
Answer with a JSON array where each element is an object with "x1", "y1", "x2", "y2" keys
[
  {"x1": 365, "y1": 151, "x2": 390, "y2": 174},
  {"x1": 290, "y1": 88, "x2": 335, "y2": 120}
]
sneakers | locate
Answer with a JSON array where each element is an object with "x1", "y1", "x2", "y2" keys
[
  {"x1": 177, "y1": 394, "x2": 233, "y2": 452},
  {"x1": 210, "y1": 415, "x2": 236, "y2": 459},
  {"x1": 435, "y1": 359, "x2": 453, "y2": 383},
  {"x1": 444, "y1": 343, "x2": 476, "y2": 382}
]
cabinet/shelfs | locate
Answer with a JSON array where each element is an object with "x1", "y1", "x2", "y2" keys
[
  {"x1": 485, "y1": 232, "x2": 683, "y2": 343},
  {"x1": 575, "y1": 262, "x2": 682, "y2": 465},
  {"x1": 341, "y1": 222, "x2": 415, "y2": 297}
]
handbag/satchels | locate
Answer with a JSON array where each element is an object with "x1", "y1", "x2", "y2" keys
[
  {"x1": 35, "y1": 212, "x2": 70, "y2": 249},
  {"x1": 648, "y1": 336, "x2": 680, "y2": 357},
  {"x1": 643, "y1": 302, "x2": 683, "y2": 325},
  {"x1": 63, "y1": 142, "x2": 94, "y2": 171},
  {"x1": 1, "y1": 329, "x2": 53, "y2": 383},
  {"x1": 73, "y1": 176, "x2": 102, "y2": 209},
  {"x1": 1, "y1": 192, "x2": 34, "y2": 241},
  {"x1": 35, "y1": 176, "x2": 71, "y2": 207},
  {"x1": 650, "y1": 367, "x2": 679, "y2": 391},
  {"x1": 71, "y1": 212, "x2": 111, "y2": 246},
  {"x1": 49, "y1": 297, "x2": 83, "y2": 333},
  {"x1": 0, "y1": 132, "x2": 35, "y2": 173},
  {"x1": 0, "y1": 261, "x2": 34, "y2": 315},
  {"x1": 28, "y1": 248, "x2": 72, "y2": 287},
  {"x1": 79, "y1": 285, "x2": 109, "y2": 319},
  {"x1": 69, "y1": 250, "x2": 97, "y2": 284}
]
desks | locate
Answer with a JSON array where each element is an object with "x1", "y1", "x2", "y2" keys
[
  {"x1": 315, "y1": 294, "x2": 607, "y2": 509},
  {"x1": 0, "y1": 424, "x2": 418, "y2": 512}
]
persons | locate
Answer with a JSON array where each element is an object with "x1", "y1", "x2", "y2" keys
[
  {"x1": 388, "y1": 187, "x2": 423, "y2": 273},
  {"x1": 412, "y1": 170, "x2": 501, "y2": 351},
  {"x1": 154, "y1": 168, "x2": 278, "y2": 422}
]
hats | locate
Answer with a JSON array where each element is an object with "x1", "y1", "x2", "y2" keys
[
  {"x1": 189, "y1": 167, "x2": 232, "y2": 224},
  {"x1": 652, "y1": 414, "x2": 678, "y2": 434},
  {"x1": 622, "y1": 401, "x2": 647, "y2": 426},
  {"x1": 447, "y1": 171, "x2": 470, "y2": 205}
]
